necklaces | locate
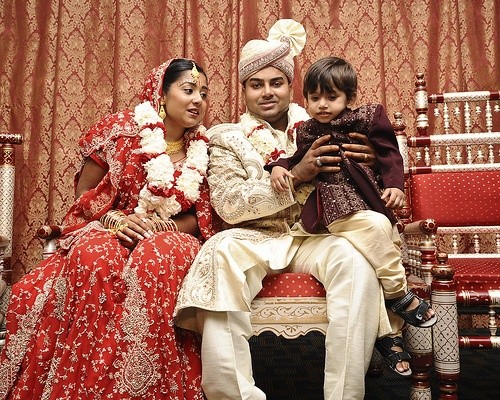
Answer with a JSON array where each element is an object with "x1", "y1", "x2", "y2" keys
[{"x1": 166, "y1": 137, "x2": 185, "y2": 156}]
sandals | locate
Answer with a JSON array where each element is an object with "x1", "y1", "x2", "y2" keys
[
  {"x1": 387, "y1": 290, "x2": 437, "y2": 329},
  {"x1": 374, "y1": 337, "x2": 413, "y2": 378}
]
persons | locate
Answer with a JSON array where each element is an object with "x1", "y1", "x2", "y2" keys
[
  {"x1": 171, "y1": 39, "x2": 393, "y2": 400},
  {"x1": 263, "y1": 56, "x2": 438, "y2": 376},
  {"x1": 0, "y1": 57, "x2": 222, "y2": 400}
]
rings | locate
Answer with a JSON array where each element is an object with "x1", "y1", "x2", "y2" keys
[
  {"x1": 364, "y1": 153, "x2": 369, "y2": 162},
  {"x1": 316, "y1": 156, "x2": 322, "y2": 167},
  {"x1": 115, "y1": 222, "x2": 131, "y2": 234}
]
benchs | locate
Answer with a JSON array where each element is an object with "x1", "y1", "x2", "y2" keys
[{"x1": 37, "y1": 220, "x2": 432, "y2": 400}]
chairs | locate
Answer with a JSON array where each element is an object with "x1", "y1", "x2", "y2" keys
[{"x1": 392, "y1": 73, "x2": 500, "y2": 400}]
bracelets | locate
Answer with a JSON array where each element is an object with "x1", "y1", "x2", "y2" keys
[{"x1": 100, "y1": 210, "x2": 179, "y2": 232}]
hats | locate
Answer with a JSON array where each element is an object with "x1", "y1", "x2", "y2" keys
[{"x1": 236, "y1": 19, "x2": 307, "y2": 84}]
